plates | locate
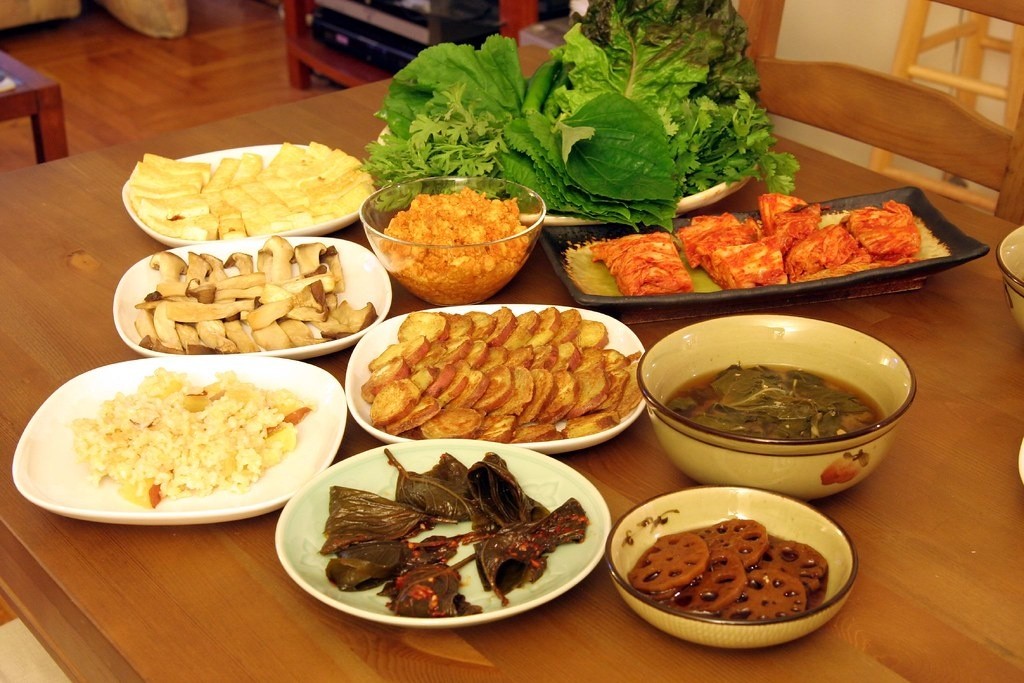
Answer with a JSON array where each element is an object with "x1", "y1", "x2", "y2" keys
[
  {"x1": 112, "y1": 234, "x2": 394, "y2": 359},
  {"x1": 9, "y1": 354, "x2": 349, "y2": 527},
  {"x1": 344, "y1": 303, "x2": 648, "y2": 453},
  {"x1": 274, "y1": 437, "x2": 613, "y2": 630},
  {"x1": 377, "y1": 121, "x2": 761, "y2": 225},
  {"x1": 121, "y1": 143, "x2": 376, "y2": 246},
  {"x1": 540, "y1": 184, "x2": 994, "y2": 311}
]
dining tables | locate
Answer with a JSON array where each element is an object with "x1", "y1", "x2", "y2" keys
[{"x1": 0, "y1": 46, "x2": 1024, "y2": 683}]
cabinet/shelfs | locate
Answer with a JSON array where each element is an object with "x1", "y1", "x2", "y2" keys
[{"x1": 286, "y1": 0, "x2": 571, "y2": 89}]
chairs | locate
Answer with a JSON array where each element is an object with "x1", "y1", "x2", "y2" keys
[{"x1": 738, "y1": 0, "x2": 1024, "y2": 227}]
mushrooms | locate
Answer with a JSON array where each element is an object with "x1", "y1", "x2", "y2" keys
[{"x1": 133, "y1": 236, "x2": 378, "y2": 354}]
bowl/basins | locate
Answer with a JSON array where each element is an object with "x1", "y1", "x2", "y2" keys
[
  {"x1": 995, "y1": 225, "x2": 1024, "y2": 332},
  {"x1": 604, "y1": 485, "x2": 859, "y2": 647},
  {"x1": 360, "y1": 175, "x2": 547, "y2": 308},
  {"x1": 637, "y1": 314, "x2": 917, "y2": 500}
]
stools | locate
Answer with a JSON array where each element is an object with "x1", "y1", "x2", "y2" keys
[{"x1": 868, "y1": 0, "x2": 1024, "y2": 213}]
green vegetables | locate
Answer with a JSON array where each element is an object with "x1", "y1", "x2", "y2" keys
[
  {"x1": 320, "y1": 448, "x2": 586, "y2": 616},
  {"x1": 360, "y1": 0, "x2": 800, "y2": 232},
  {"x1": 667, "y1": 364, "x2": 873, "y2": 440}
]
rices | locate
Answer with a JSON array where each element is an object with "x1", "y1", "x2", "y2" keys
[{"x1": 66, "y1": 366, "x2": 314, "y2": 508}]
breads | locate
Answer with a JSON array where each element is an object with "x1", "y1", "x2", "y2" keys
[{"x1": 361, "y1": 308, "x2": 643, "y2": 443}]
